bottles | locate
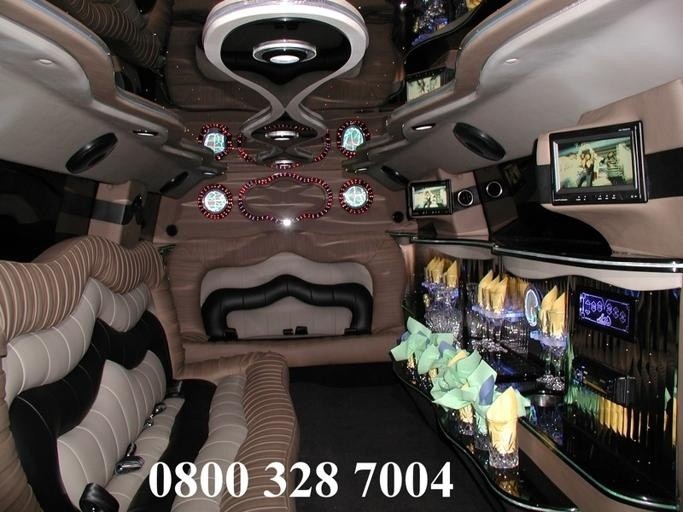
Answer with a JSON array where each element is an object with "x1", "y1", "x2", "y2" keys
[
  {"x1": 420, "y1": 280, "x2": 480, "y2": 358},
  {"x1": 435, "y1": 0, "x2": 449, "y2": 32}
]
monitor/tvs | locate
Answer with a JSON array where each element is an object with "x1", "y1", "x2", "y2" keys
[
  {"x1": 407, "y1": 178, "x2": 453, "y2": 219},
  {"x1": 549, "y1": 120, "x2": 648, "y2": 206}
]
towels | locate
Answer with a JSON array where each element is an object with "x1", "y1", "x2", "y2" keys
[{"x1": 389, "y1": 256, "x2": 567, "y2": 468}]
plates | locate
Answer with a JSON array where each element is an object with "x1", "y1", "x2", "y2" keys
[{"x1": 523, "y1": 284, "x2": 541, "y2": 331}]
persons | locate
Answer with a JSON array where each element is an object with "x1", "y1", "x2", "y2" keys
[
  {"x1": 423, "y1": 191, "x2": 444, "y2": 208},
  {"x1": 576, "y1": 151, "x2": 625, "y2": 187}
]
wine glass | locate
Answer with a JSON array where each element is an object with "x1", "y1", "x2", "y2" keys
[{"x1": 534, "y1": 333, "x2": 567, "y2": 392}]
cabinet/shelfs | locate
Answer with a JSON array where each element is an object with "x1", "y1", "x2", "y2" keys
[{"x1": 387, "y1": 231, "x2": 682, "y2": 512}]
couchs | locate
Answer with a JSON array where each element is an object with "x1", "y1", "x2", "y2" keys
[
  {"x1": 152, "y1": 227, "x2": 405, "y2": 367},
  {"x1": 1, "y1": 236, "x2": 301, "y2": 512}
]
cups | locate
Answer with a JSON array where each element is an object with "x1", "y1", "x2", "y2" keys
[
  {"x1": 403, "y1": 353, "x2": 520, "y2": 470},
  {"x1": 477, "y1": 310, "x2": 530, "y2": 360}
]
